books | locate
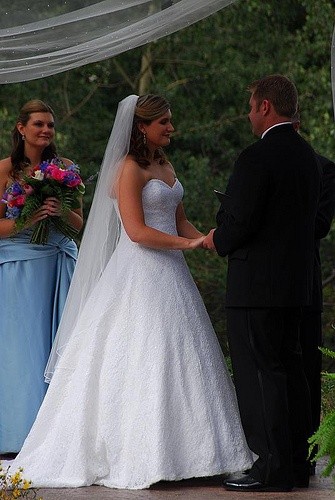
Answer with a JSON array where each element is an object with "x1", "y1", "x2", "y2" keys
[{"x1": 214, "y1": 191, "x2": 224, "y2": 202}]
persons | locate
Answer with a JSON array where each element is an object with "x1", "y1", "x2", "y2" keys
[
  {"x1": 105, "y1": 94, "x2": 207, "y2": 493},
  {"x1": 201, "y1": 73, "x2": 335, "y2": 491},
  {"x1": 0, "y1": 98, "x2": 85, "y2": 456}
]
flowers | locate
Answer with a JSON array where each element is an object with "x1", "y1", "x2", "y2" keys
[
  {"x1": 0, "y1": 154, "x2": 81, "y2": 246},
  {"x1": 0, "y1": 461, "x2": 43, "y2": 500}
]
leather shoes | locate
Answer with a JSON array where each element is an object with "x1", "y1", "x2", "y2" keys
[{"x1": 224, "y1": 469, "x2": 310, "y2": 492}]
